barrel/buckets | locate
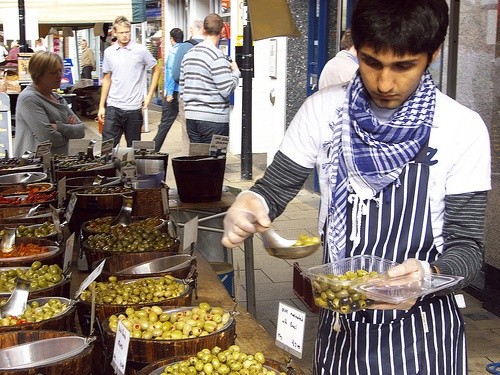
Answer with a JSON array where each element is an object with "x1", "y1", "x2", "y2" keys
[
  {"x1": 134, "y1": 353, "x2": 297, "y2": 375},
  {"x1": 81, "y1": 216, "x2": 167, "y2": 240},
  {"x1": 0, "y1": 330, "x2": 94, "y2": 375},
  {"x1": 0, "y1": 296, "x2": 77, "y2": 332},
  {"x1": 0, "y1": 172, "x2": 47, "y2": 185},
  {"x1": 0, "y1": 183, "x2": 56, "y2": 197},
  {"x1": 0, "y1": 223, "x2": 65, "y2": 246},
  {"x1": 0, "y1": 237, "x2": 65, "y2": 269},
  {"x1": 76, "y1": 277, "x2": 193, "y2": 335},
  {"x1": 66, "y1": 186, "x2": 138, "y2": 226},
  {"x1": 91, "y1": 252, "x2": 194, "y2": 289},
  {"x1": 209, "y1": 262, "x2": 234, "y2": 297},
  {"x1": 57, "y1": 176, "x2": 123, "y2": 191},
  {"x1": 0, "y1": 207, "x2": 66, "y2": 224},
  {"x1": 102, "y1": 305, "x2": 237, "y2": 375},
  {"x1": 0, "y1": 267, "x2": 71, "y2": 300},
  {"x1": 0, "y1": 195, "x2": 67, "y2": 208},
  {"x1": 171, "y1": 155, "x2": 226, "y2": 203},
  {"x1": 54, "y1": 163, "x2": 116, "y2": 181},
  {"x1": 82, "y1": 236, "x2": 181, "y2": 276}
]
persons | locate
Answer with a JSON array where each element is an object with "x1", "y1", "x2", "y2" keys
[
  {"x1": 220, "y1": 0, "x2": 490, "y2": 375},
  {"x1": 12, "y1": 51, "x2": 85, "y2": 157},
  {"x1": 97, "y1": 17, "x2": 162, "y2": 157},
  {"x1": 0, "y1": 34, "x2": 46, "y2": 67},
  {"x1": 80, "y1": 40, "x2": 96, "y2": 80},
  {"x1": 178, "y1": 13, "x2": 240, "y2": 191},
  {"x1": 150, "y1": 28, "x2": 197, "y2": 154},
  {"x1": 315, "y1": 26, "x2": 361, "y2": 246}
]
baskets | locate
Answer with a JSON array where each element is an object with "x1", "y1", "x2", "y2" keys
[{"x1": 126, "y1": 180, "x2": 170, "y2": 222}]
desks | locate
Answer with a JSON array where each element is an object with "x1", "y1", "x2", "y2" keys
[{"x1": 66, "y1": 178, "x2": 306, "y2": 375}]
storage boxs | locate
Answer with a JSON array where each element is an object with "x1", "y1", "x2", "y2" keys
[{"x1": 308, "y1": 254, "x2": 464, "y2": 315}]
